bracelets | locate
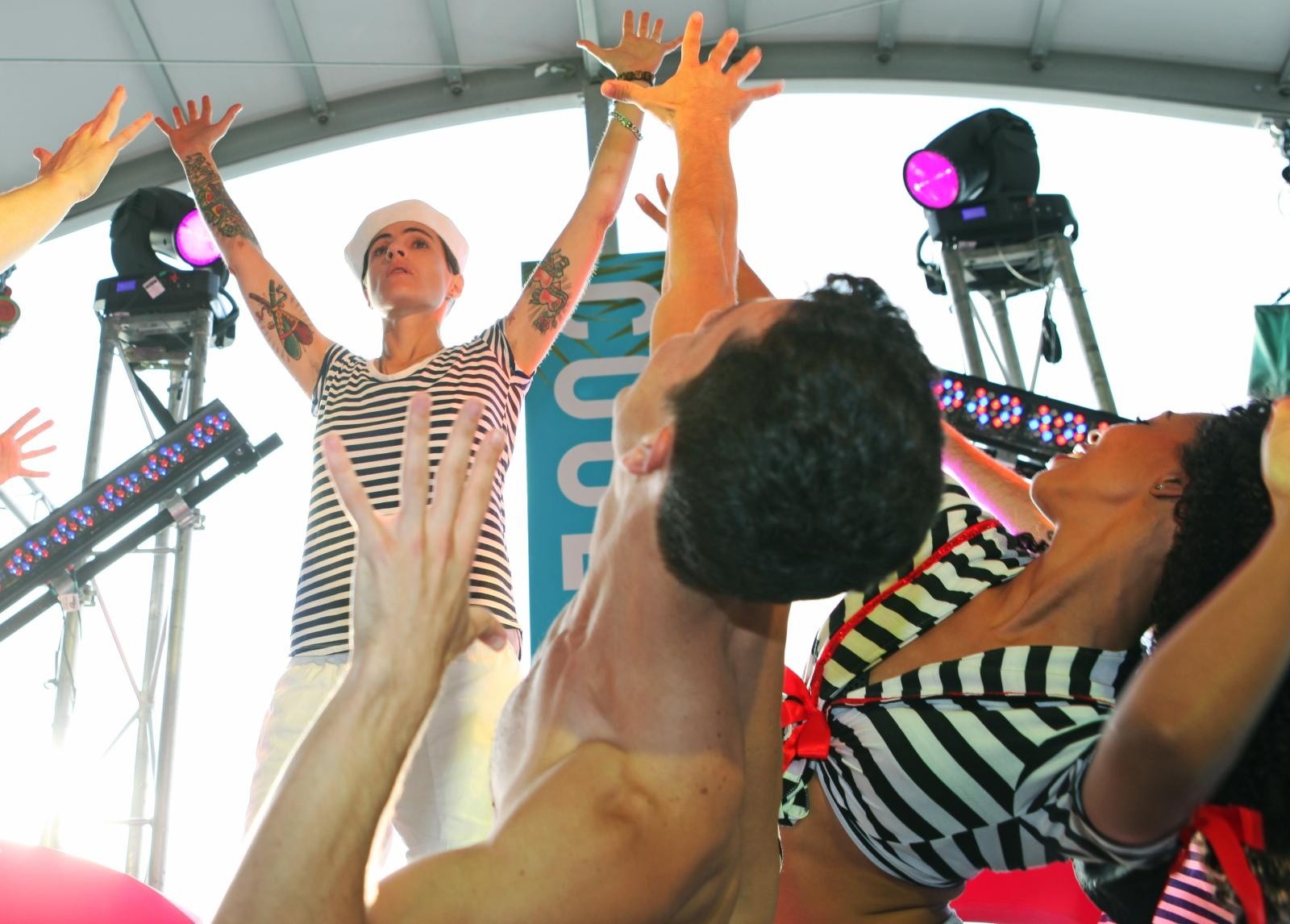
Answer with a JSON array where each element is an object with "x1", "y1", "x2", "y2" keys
[{"x1": 610, "y1": 70, "x2": 656, "y2": 141}]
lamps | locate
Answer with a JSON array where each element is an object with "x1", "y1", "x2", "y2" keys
[
  {"x1": 110, "y1": 187, "x2": 228, "y2": 290},
  {"x1": 902, "y1": 107, "x2": 1042, "y2": 212}
]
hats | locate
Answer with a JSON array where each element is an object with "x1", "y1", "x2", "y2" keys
[{"x1": 342, "y1": 199, "x2": 470, "y2": 280}]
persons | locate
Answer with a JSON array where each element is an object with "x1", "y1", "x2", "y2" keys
[
  {"x1": 0, "y1": 87, "x2": 154, "y2": 482},
  {"x1": 154, "y1": 9, "x2": 683, "y2": 863},
  {"x1": 219, "y1": 10, "x2": 945, "y2": 924},
  {"x1": 635, "y1": 173, "x2": 1290, "y2": 924}
]
